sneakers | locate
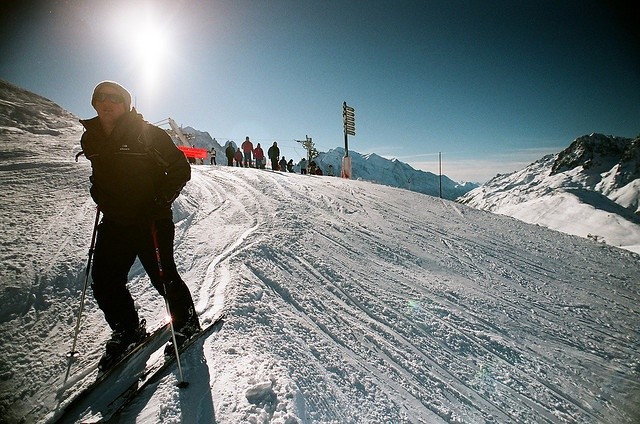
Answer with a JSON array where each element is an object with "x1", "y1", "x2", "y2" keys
[
  {"x1": 105, "y1": 320, "x2": 142, "y2": 353},
  {"x1": 170, "y1": 314, "x2": 203, "y2": 343}
]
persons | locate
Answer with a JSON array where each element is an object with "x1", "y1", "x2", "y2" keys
[
  {"x1": 74, "y1": 80, "x2": 201, "y2": 371},
  {"x1": 287, "y1": 159, "x2": 295, "y2": 173},
  {"x1": 207, "y1": 148, "x2": 217, "y2": 165},
  {"x1": 253, "y1": 143, "x2": 263, "y2": 168},
  {"x1": 309, "y1": 161, "x2": 316, "y2": 174},
  {"x1": 314, "y1": 166, "x2": 322, "y2": 175},
  {"x1": 268, "y1": 142, "x2": 279, "y2": 170},
  {"x1": 242, "y1": 136, "x2": 254, "y2": 168},
  {"x1": 278, "y1": 156, "x2": 287, "y2": 171},
  {"x1": 234, "y1": 148, "x2": 243, "y2": 167},
  {"x1": 327, "y1": 165, "x2": 334, "y2": 176},
  {"x1": 261, "y1": 155, "x2": 266, "y2": 167},
  {"x1": 226, "y1": 142, "x2": 235, "y2": 166},
  {"x1": 297, "y1": 158, "x2": 308, "y2": 174}
]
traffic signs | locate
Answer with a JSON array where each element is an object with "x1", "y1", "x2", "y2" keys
[
  {"x1": 347, "y1": 126, "x2": 355, "y2": 131},
  {"x1": 347, "y1": 116, "x2": 355, "y2": 121},
  {"x1": 346, "y1": 111, "x2": 355, "y2": 116},
  {"x1": 346, "y1": 106, "x2": 355, "y2": 112},
  {"x1": 347, "y1": 121, "x2": 356, "y2": 126},
  {"x1": 347, "y1": 131, "x2": 356, "y2": 136}
]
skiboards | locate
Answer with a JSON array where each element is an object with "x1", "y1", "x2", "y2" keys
[{"x1": 36, "y1": 312, "x2": 225, "y2": 424}]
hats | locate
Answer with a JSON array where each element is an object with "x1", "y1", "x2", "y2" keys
[{"x1": 91, "y1": 81, "x2": 131, "y2": 107}]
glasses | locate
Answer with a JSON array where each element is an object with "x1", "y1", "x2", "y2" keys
[{"x1": 93, "y1": 91, "x2": 126, "y2": 104}]
